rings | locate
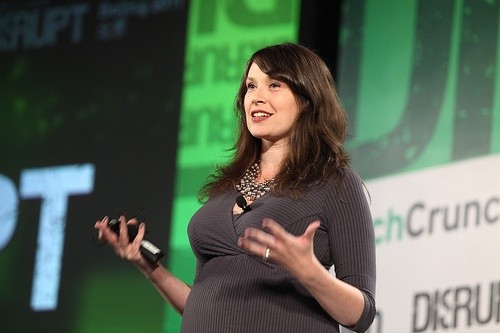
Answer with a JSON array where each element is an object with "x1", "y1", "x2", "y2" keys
[{"x1": 265, "y1": 246, "x2": 271, "y2": 260}]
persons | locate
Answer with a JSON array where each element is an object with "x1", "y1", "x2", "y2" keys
[{"x1": 94, "y1": 41, "x2": 377, "y2": 333}]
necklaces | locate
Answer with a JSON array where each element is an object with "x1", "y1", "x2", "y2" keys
[{"x1": 236, "y1": 158, "x2": 276, "y2": 201}]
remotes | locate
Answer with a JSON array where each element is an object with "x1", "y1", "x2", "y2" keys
[{"x1": 109, "y1": 219, "x2": 165, "y2": 264}]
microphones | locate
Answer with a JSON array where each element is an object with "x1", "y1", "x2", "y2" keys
[{"x1": 236, "y1": 196, "x2": 251, "y2": 212}]
